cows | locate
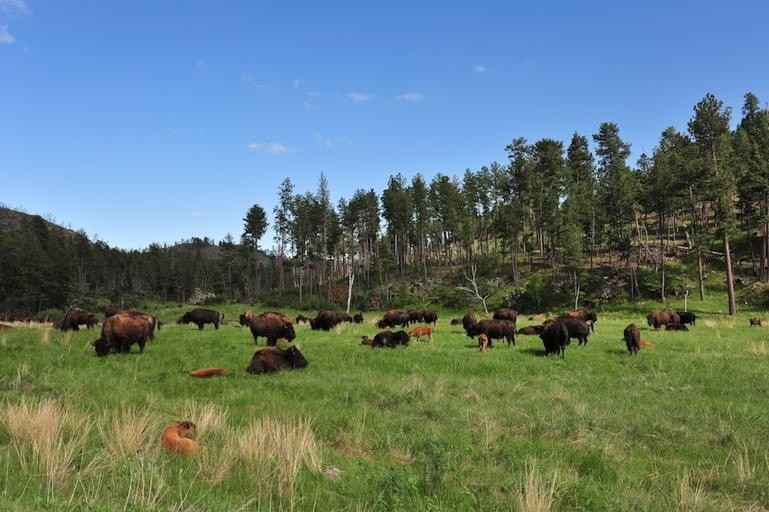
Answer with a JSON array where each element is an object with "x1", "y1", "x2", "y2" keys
[
  {"x1": 175, "y1": 307, "x2": 226, "y2": 330},
  {"x1": 160, "y1": 418, "x2": 202, "y2": 457},
  {"x1": 92, "y1": 306, "x2": 161, "y2": 356},
  {"x1": 188, "y1": 367, "x2": 228, "y2": 378},
  {"x1": 240, "y1": 296, "x2": 440, "y2": 348},
  {"x1": 645, "y1": 307, "x2": 697, "y2": 331},
  {"x1": 245, "y1": 344, "x2": 309, "y2": 377},
  {"x1": 621, "y1": 322, "x2": 641, "y2": 355},
  {"x1": 59, "y1": 307, "x2": 97, "y2": 331},
  {"x1": 448, "y1": 307, "x2": 598, "y2": 360}
]
